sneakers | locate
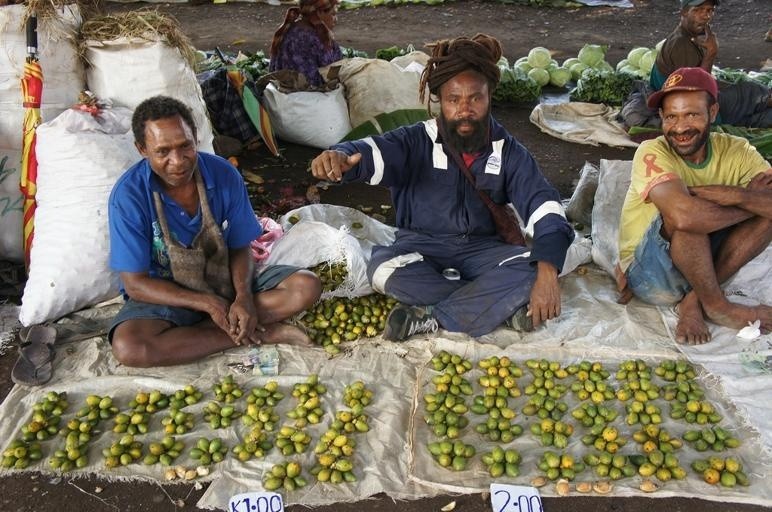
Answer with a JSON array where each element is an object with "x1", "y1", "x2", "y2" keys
[
  {"x1": 381, "y1": 301, "x2": 438, "y2": 342},
  {"x1": 503, "y1": 304, "x2": 536, "y2": 332}
]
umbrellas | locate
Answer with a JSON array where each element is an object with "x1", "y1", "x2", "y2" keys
[{"x1": 17, "y1": 11, "x2": 44, "y2": 275}]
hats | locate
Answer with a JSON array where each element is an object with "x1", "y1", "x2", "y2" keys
[
  {"x1": 644, "y1": 67, "x2": 720, "y2": 111},
  {"x1": 679, "y1": 0, "x2": 719, "y2": 9}
]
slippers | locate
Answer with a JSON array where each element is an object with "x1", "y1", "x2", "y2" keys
[{"x1": 10, "y1": 315, "x2": 115, "y2": 388}]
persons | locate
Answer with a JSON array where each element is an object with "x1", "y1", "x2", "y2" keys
[
  {"x1": 108, "y1": 96, "x2": 322, "y2": 366},
  {"x1": 311, "y1": 33, "x2": 575, "y2": 341},
  {"x1": 270, "y1": 0, "x2": 342, "y2": 86},
  {"x1": 619, "y1": 67, "x2": 771, "y2": 344},
  {"x1": 651, "y1": 0, "x2": 772, "y2": 129}
]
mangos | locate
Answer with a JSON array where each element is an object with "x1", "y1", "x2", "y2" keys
[
  {"x1": 1, "y1": 375, "x2": 373, "y2": 493},
  {"x1": 302, "y1": 258, "x2": 398, "y2": 356},
  {"x1": 421, "y1": 349, "x2": 752, "y2": 490}
]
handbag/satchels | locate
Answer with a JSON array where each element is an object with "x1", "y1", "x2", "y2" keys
[
  {"x1": 167, "y1": 230, "x2": 236, "y2": 304},
  {"x1": 489, "y1": 199, "x2": 527, "y2": 248}
]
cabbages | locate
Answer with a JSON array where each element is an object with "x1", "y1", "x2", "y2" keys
[{"x1": 499, "y1": 37, "x2": 666, "y2": 88}]
